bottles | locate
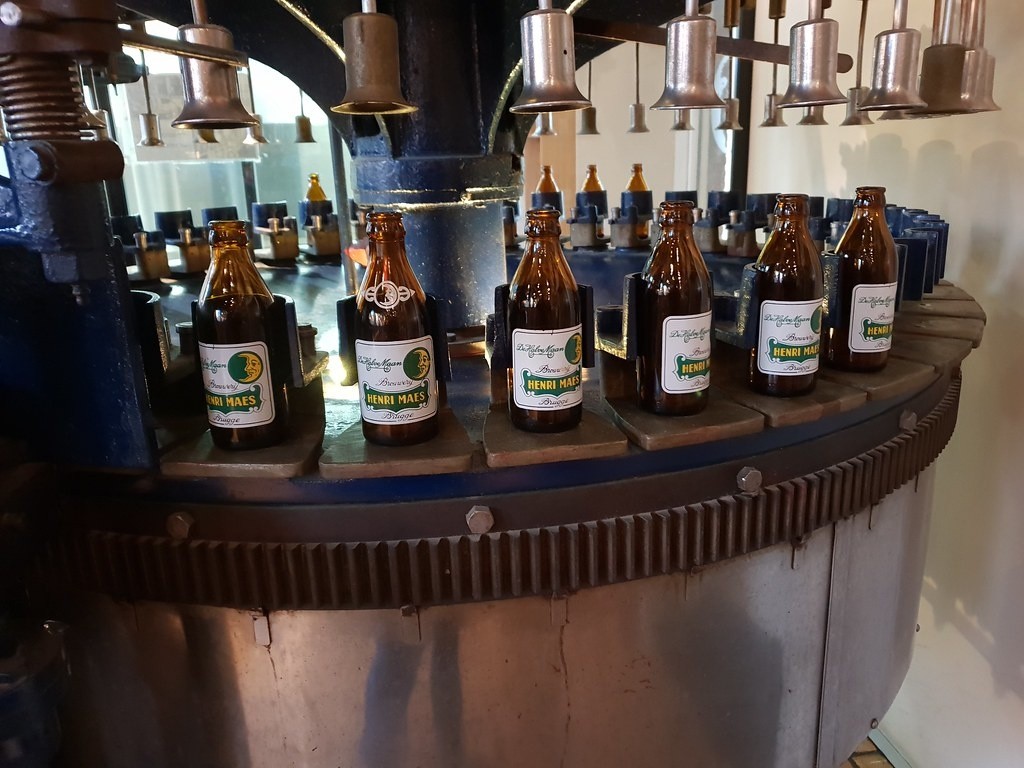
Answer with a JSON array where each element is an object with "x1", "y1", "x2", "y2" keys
[
  {"x1": 535, "y1": 165, "x2": 559, "y2": 193},
  {"x1": 353, "y1": 209, "x2": 439, "y2": 447},
  {"x1": 582, "y1": 164, "x2": 606, "y2": 237},
  {"x1": 306, "y1": 173, "x2": 327, "y2": 202},
  {"x1": 634, "y1": 201, "x2": 712, "y2": 416},
  {"x1": 625, "y1": 163, "x2": 649, "y2": 238},
  {"x1": 195, "y1": 220, "x2": 290, "y2": 451},
  {"x1": 746, "y1": 193, "x2": 825, "y2": 393},
  {"x1": 506, "y1": 207, "x2": 583, "y2": 434},
  {"x1": 828, "y1": 183, "x2": 898, "y2": 374}
]
